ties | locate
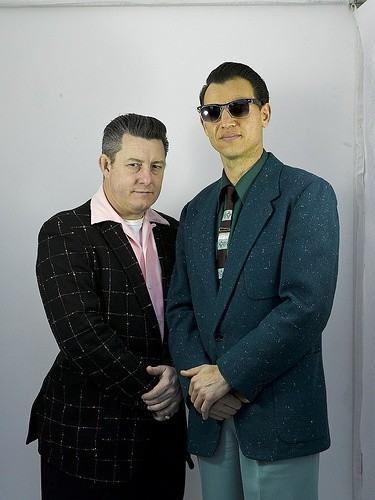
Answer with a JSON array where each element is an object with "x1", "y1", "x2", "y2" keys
[{"x1": 217, "y1": 185, "x2": 236, "y2": 284}]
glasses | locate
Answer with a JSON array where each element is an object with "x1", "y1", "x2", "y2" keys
[{"x1": 198, "y1": 98, "x2": 262, "y2": 121}]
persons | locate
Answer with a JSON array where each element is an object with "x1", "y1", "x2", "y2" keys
[
  {"x1": 25, "y1": 114, "x2": 194, "y2": 500},
  {"x1": 166, "y1": 62, "x2": 340, "y2": 500}
]
